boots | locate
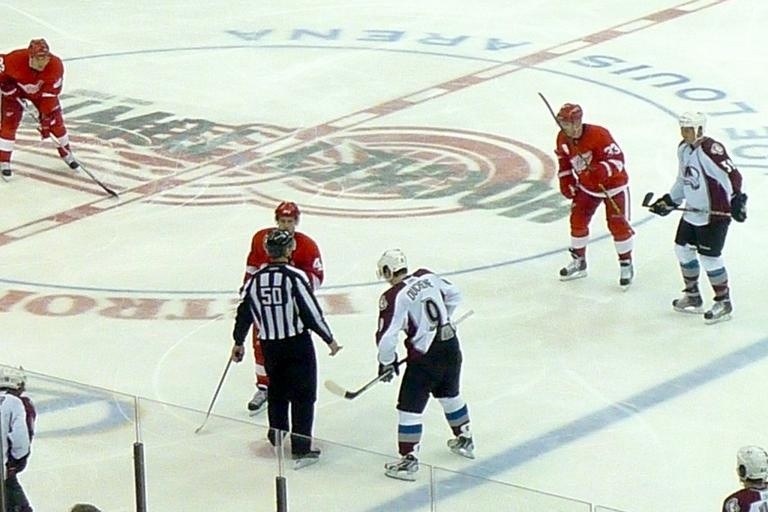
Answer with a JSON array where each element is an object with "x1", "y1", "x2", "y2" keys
[
  {"x1": 248, "y1": 383, "x2": 474, "y2": 473},
  {"x1": 560, "y1": 247, "x2": 732, "y2": 319}
]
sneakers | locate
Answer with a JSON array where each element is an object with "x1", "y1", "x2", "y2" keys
[
  {"x1": 1, "y1": 162, "x2": 10, "y2": 176},
  {"x1": 62, "y1": 153, "x2": 78, "y2": 169}
]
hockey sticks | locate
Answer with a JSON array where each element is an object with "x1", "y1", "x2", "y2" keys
[
  {"x1": 16, "y1": 97, "x2": 119, "y2": 196},
  {"x1": 642, "y1": 192, "x2": 733, "y2": 217},
  {"x1": 324, "y1": 310, "x2": 474, "y2": 399},
  {"x1": 538, "y1": 91, "x2": 634, "y2": 234},
  {"x1": 194, "y1": 354, "x2": 233, "y2": 432}
]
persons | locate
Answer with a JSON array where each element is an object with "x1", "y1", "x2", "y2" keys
[
  {"x1": 556, "y1": 102, "x2": 634, "y2": 290},
  {"x1": 722, "y1": 445, "x2": 768, "y2": 512},
  {"x1": 237, "y1": 201, "x2": 324, "y2": 413},
  {"x1": 0, "y1": 363, "x2": 36, "y2": 512},
  {"x1": 0, "y1": 38, "x2": 81, "y2": 177},
  {"x1": 229, "y1": 229, "x2": 339, "y2": 458},
  {"x1": 651, "y1": 111, "x2": 747, "y2": 324},
  {"x1": 374, "y1": 246, "x2": 474, "y2": 473}
]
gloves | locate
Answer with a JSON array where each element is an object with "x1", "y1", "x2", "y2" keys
[
  {"x1": 648, "y1": 194, "x2": 678, "y2": 215},
  {"x1": 41, "y1": 119, "x2": 50, "y2": 137},
  {"x1": 1, "y1": 83, "x2": 21, "y2": 101},
  {"x1": 730, "y1": 192, "x2": 746, "y2": 222},
  {"x1": 557, "y1": 169, "x2": 575, "y2": 199},
  {"x1": 378, "y1": 352, "x2": 398, "y2": 382},
  {"x1": 579, "y1": 165, "x2": 607, "y2": 185}
]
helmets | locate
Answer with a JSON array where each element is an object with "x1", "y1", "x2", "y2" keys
[
  {"x1": 261, "y1": 201, "x2": 407, "y2": 281},
  {"x1": 0, "y1": 365, "x2": 26, "y2": 390},
  {"x1": 736, "y1": 446, "x2": 767, "y2": 481},
  {"x1": 28, "y1": 38, "x2": 48, "y2": 58},
  {"x1": 678, "y1": 111, "x2": 706, "y2": 127},
  {"x1": 556, "y1": 103, "x2": 581, "y2": 122}
]
